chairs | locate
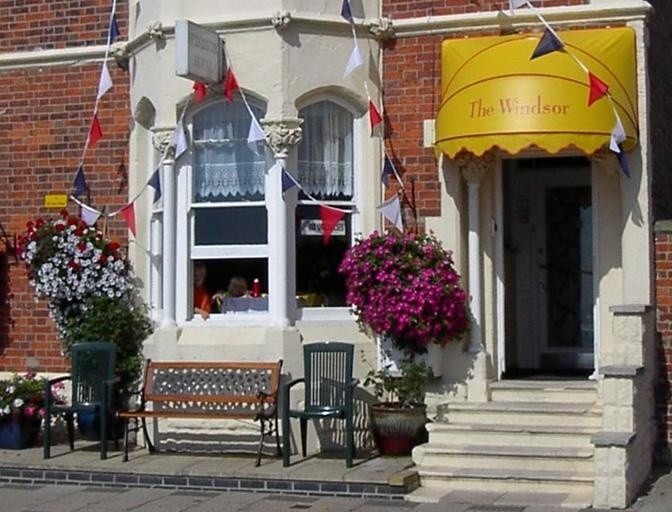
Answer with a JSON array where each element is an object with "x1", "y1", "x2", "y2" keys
[
  {"x1": 44, "y1": 341, "x2": 121, "y2": 460},
  {"x1": 282, "y1": 342, "x2": 360, "y2": 468}
]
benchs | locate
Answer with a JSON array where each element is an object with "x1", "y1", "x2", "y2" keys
[{"x1": 115, "y1": 359, "x2": 283, "y2": 467}]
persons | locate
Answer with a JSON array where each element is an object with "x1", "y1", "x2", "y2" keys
[
  {"x1": 193, "y1": 259, "x2": 213, "y2": 321},
  {"x1": 222, "y1": 276, "x2": 249, "y2": 298}
]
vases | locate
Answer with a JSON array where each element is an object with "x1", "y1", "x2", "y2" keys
[
  {"x1": 0, "y1": 418, "x2": 41, "y2": 450},
  {"x1": 376, "y1": 330, "x2": 441, "y2": 378}
]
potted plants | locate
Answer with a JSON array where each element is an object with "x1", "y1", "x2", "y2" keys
[
  {"x1": 360, "y1": 349, "x2": 434, "y2": 456},
  {"x1": 60, "y1": 294, "x2": 153, "y2": 441}
]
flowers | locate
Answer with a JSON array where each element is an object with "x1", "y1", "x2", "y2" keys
[
  {"x1": 337, "y1": 225, "x2": 473, "y2": 352},
  {"x1": 0, "y1": 368, "x2": 65, "y2": 419},
  {"x1": 14, "y1": 208, "x2": 133, "y2": 348}
]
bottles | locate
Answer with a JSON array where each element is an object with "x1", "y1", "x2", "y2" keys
[{"x1": 253, "y1": 278, "x2": 260, "y2": 297}]
